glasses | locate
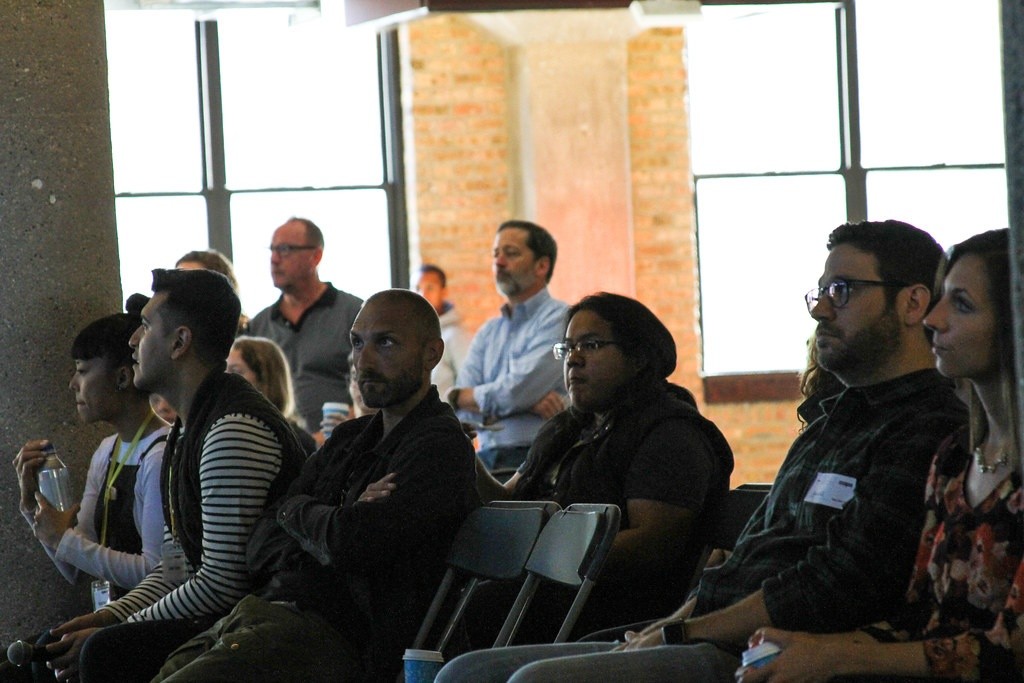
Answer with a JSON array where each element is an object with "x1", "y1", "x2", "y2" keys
[
  {"x1": 553, "y1": 341, "x2": 641, "y2": 360},
  {"x1": 269, "y1": 244, "x2": 317, "y2": 255},
  {"x1": 805, "y1": 277, "x2": 916, "y2": 314}
]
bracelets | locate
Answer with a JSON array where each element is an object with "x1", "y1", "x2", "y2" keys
[{"x1": 662, "y1": 619, "x2": 688, "y2": 647}]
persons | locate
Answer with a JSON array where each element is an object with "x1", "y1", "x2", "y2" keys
[
  {"x1": 410, "y1": 262, "x2": 474, "y2": 404},
  {"x1": 734, "y1": 228, "x2": 1024, "y2": 683},
  {"x1": 223, "y1": 335, "x2": 317, "y2": 460},
  {"x1": 237, "y1": 218, "x2": 366, "y2": 436},
  {"x1": 46, "y1": 268, "x2": 309, "y2": 683},
  {"x1": 442, "y1": 220, "x2": 575, "y2": 486},
  {"x1": 149, "y1": 289, "x2": 479, "y2": 683},
  {"x1": 502, "y1": 291, "x2": 736, "y2": 535},
  {"x1": 0, "y1": 313, "x2": 176, "y2": 683},
  {"x1": 432, "y1": 219, "x2": 970, "y2": 683},
  {"x1": 175, "y1": 248, "x2": 248, "y2": 337}
]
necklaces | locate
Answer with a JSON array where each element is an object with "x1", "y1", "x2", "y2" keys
[
  {"x1": 583, "y1": 408, "x2": 614, "y2": 445},
  {"x1": 975, "y1": 435, "x2": 1009, "y2": 475}
]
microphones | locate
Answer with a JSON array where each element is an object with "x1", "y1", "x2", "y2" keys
[{"x1": 7, "y1": 640, "x2": 70, "y2": 667}]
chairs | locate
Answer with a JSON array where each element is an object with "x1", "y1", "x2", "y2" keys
[{"x1": 392, "y1": 484, "x2": 782, "y2": 683}]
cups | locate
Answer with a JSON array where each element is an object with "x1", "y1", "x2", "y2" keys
[
  {"x1": 403, "y1": 649, "x2": 444, "y2": 683},
  {"x1": 742, "y1": 642, "x2": 782, "y2": 669},
  {"x1": 322, "y1": 402, "x2": 349, "y2": 438}
]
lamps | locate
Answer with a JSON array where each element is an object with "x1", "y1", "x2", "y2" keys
[{"x1": 629, "y1": 1, "x2": 702, "y2": 27}]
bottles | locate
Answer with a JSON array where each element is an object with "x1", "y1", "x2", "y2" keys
[{"x1": 37, "y1": 440, "x2": 73, "y2": 512}]
